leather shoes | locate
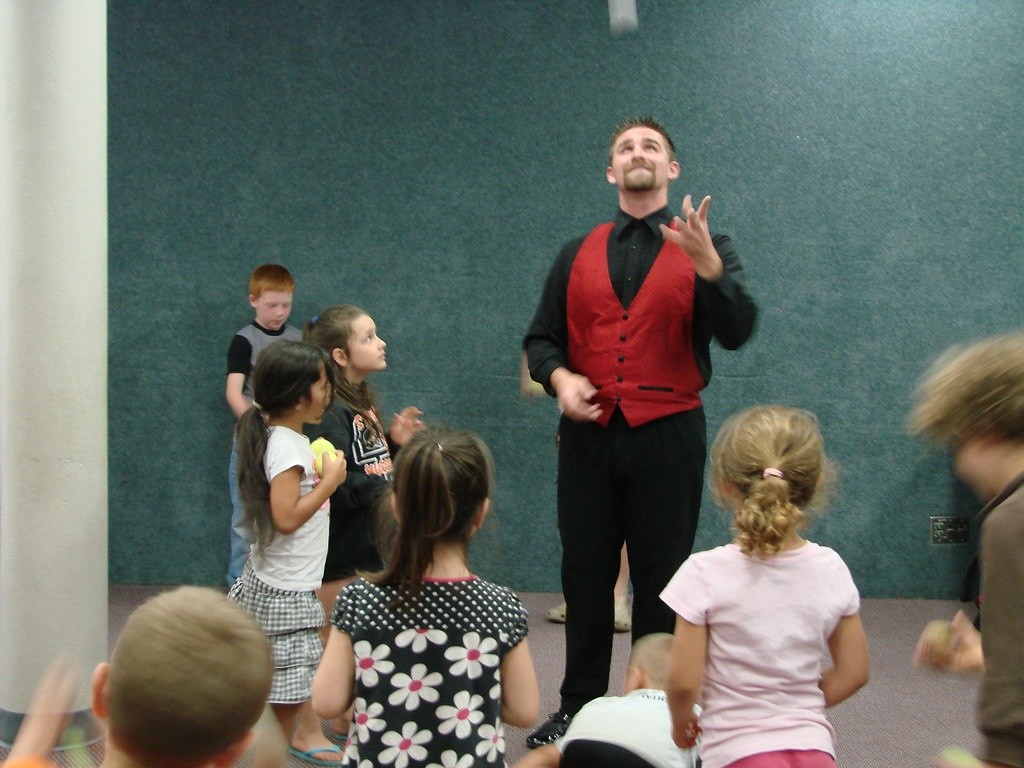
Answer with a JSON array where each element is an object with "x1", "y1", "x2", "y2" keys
[{"x1": 527, "y1": 711, "x2": 572, "y2": 748}]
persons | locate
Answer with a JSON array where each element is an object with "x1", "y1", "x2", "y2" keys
[
  {"x1": 656, "y1": 406, "x2": 875, "y2": 766},
  {"x1": 223, "y1": 262, "x2": 305, "y2": 591},
  {"x1": 3, "y1": 585, "x2": 278, "y2": 767},
  {"x1": 513, "y1": 346, "x2": 636, "y2": 631},
  {"x1": 521, "y1": 113, "x2": 758, "y2": 750},
  {"x1": 908, "y1": 334, "x2": 1022, "y2": 766},
  {"x1": 307, "y1": 429, "x2": 542, "y2": 767},
  {"x1": 220, "y1": 337, "x2": 349, "y2": 739},
  {"x1": 286, "y1": 304, "x2": 428, "y2": 766},
  {"x1": 505, "y1": 632, "x2": 705, "y2": 768}
]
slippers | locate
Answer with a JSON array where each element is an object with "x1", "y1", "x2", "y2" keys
[
  {"x1": 288, "y1": 744, "x2": 345, "y2": 766},
  {"x1": 335, "y1": 734, "x2": 347, "y2": 740}
]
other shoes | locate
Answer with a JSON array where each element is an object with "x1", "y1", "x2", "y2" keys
[
  {"x1": 614, "y1": 608, "x2": 631, "y2": 631},
  {"x1": 546, "y1": 601, "x2": 566, "y2": 623}
]
